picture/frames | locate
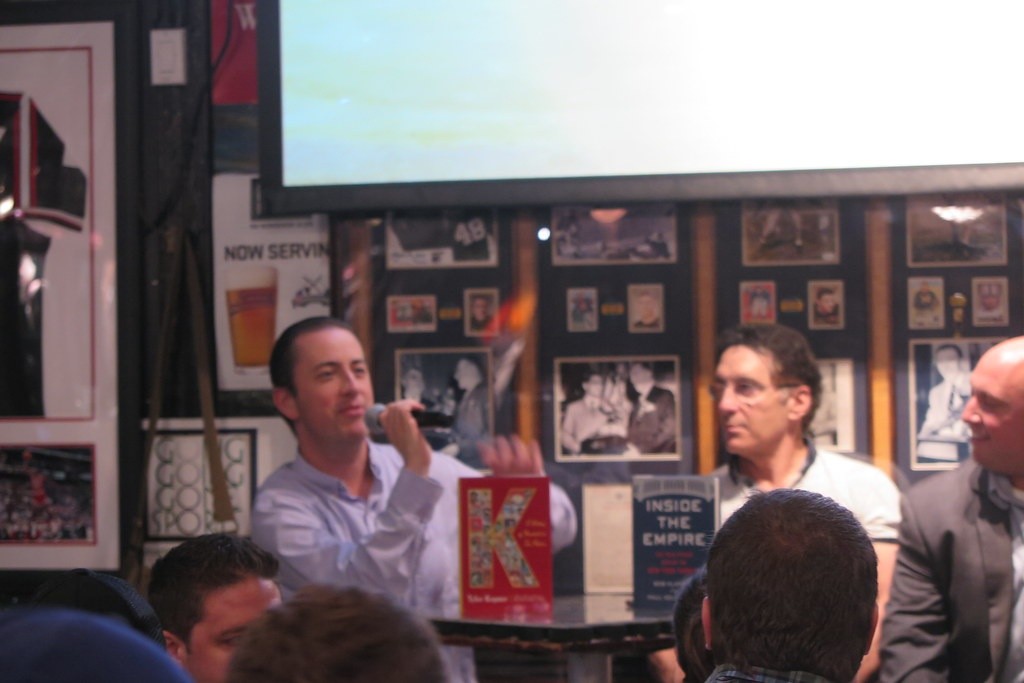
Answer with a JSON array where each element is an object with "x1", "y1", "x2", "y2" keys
[
  {"x1": 382, "y1": 185, "x2": 1012, "y2": 337},
  {"x1": 552, "y1": 355, "x2": 683, "y2": 461},
  {"x1": 907, "y1": 338, "x2": 1011, "y2": 472},
  {"x1": 0, "y1": 20, "x2": 124, "y2": 579},
  {"x1": 796, "y1": 354, "x2": 854, "y2": 452},
  {"x1": 393, "y1": 347, "x2": 494, "y2": 446}
]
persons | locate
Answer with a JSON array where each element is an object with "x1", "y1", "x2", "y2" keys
[
  {"x1": 879, "y1": 336, "x2": 1023, "y2": 683},
  {"x1": 703, "y1": 488, "x2": 878, "y2": 683},
  {"x1": 633, "y1": 289, "x2": 659, "y2": 329},
  {"x1": 919, "y1": 343, "x2": 974, "y2": 442},
  {"x1": 250, "y1": 316, "x2": 577, "y2": 683},
  {"x1": 469, "y1": 296, "x2": 489, "y2": 328},
  {"x1": 561, "y1": 362, "x2": 675, "y2": 455},
  {"x1": 0, "y1": 533, "x2": 444, "y2": 683},
  {"x1": 814, "y1": 287, "x2": 840, "y2": 324},
  {"x1": 647, "y1": 323, "x2": 903, "y2": 683},
  {"x1": 0, "y1": 461, "x2": 92, "y2": 541},
  {"x1": 400, "y1": 364, "x2": 435, "y2": 408},
  {"x1": 748, "y1": 286, "x2": 770, "y2": 318},
  {"x1": 571, "y1": 291, "x2": 592, "y2": 323},
  {"x1": 448, "y1": 352, "x2": 489, "y2": 438}
]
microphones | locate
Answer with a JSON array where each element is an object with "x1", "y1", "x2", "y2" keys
[{"x1": 362, "y1": 402, "x2": 456, "y2": 434}]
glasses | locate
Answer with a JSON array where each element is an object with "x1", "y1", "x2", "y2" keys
[{"x1": 709, "y1": 377, "x2": 792, "y2": 405}]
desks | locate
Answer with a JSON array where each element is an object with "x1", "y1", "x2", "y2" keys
[{"x1": 414, "y1": 593, "x2": 678, "y2": 683}]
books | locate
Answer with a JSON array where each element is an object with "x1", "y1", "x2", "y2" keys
[
  {"x1": 631, "y1": 474, "x2": 719, "y2": 620},
  {"x1": 457, "y1": 475, "x2": 553, "y2": 617}
]
